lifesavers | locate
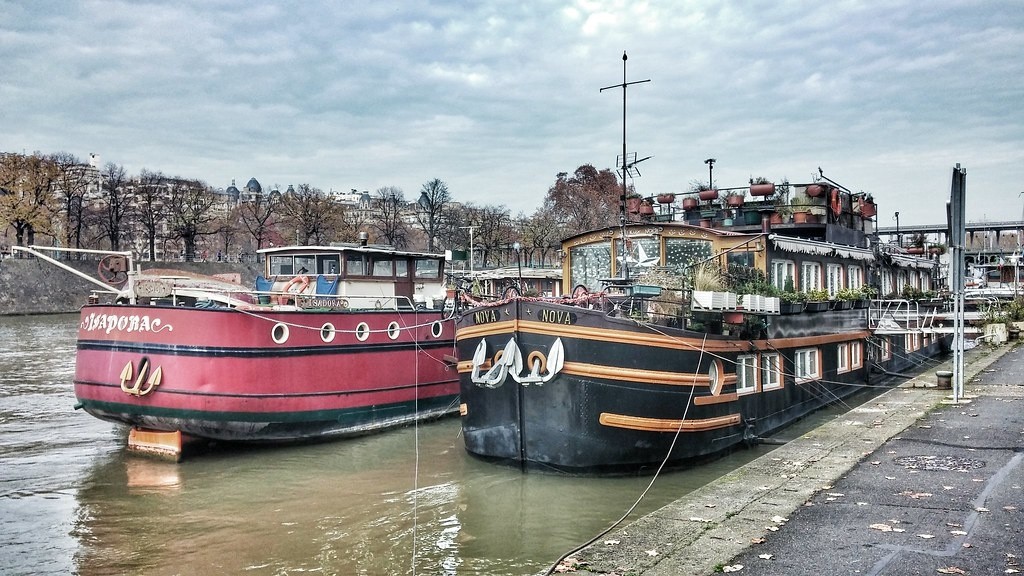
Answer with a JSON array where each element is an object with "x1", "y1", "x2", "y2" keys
[{"x1": 281, "y1": 276, "x2": 309, "y2": 299}]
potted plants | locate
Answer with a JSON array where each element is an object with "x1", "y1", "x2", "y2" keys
[
  {"x1": 860, "y1": 192, "x2": 876, "y2": 217},
  {"x1": 683, "y1": 196, "x2": 697, "y2": 210},
  {"x1": 699, "y1": 205, "x2": 734, "y2": 228},
  {"x1": 695, "y1": 179, "x2": 720, "y2": 201},
  {"x1": 806, "y1": 171, "x2": 825, "y2": 197},
  {"x1": 884, "y1": 284, "x2": 939, "y2": 306},
  {"x1": 750, "y1": 177, "x2": 776, "y2": 195},
  {"x1": 900, "y1": 231, "x2": 949, "y2": 255},
  {"x1": 637, "y1": 197, "x2": 655, "y2": 215},
  {"x1": 628, "y1": 258, "x2": 781, "y2": 341},
  {"x1": 781, "y1": 283, "x2": 876, "y2": 315},
  {"x1": 626, "y1": 192, "x2": 641, "y2": 214},
  {"x1": 975, "y1": 306, "x2": 1008, "y2": 342},
  {"x1": 721, "y1": 189, "x2": 746, "y2": 205},
  {"x1": 743, "y1": 205, "x2": 819, "y2": 225},
  {"x1": 655, "y1": 192, "x2": 675, "y2": 203}
]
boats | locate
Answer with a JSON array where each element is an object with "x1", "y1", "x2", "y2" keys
[
  {"x1": 74, "y1": 243, "x2": 462, "y2": 445},
  {"x1": 455, "y1": 49, "x2": 954, "y2": 477},
  {"x1": 963, "y1": 256, "x2": 1024, "y2": 299}
]
